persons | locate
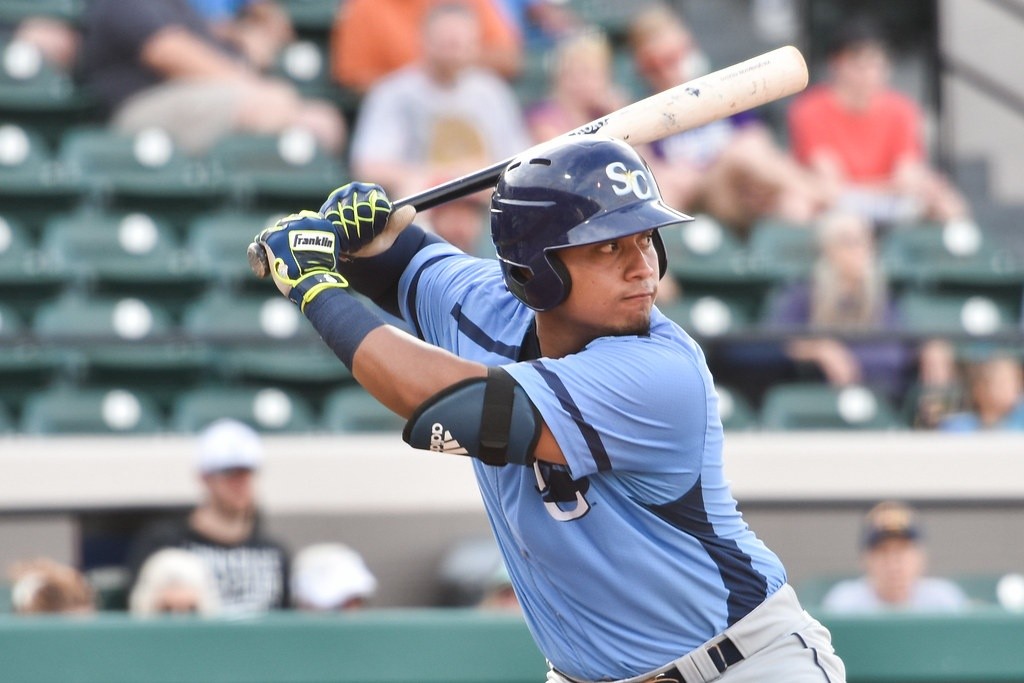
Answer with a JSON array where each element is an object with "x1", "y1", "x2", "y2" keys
[
  {"x1": 8, "y1": 420, "x2": 524, "y2": 612},
  {"x1": 1, "y1": 0, "x2": 965, "y2": 246},
  {"x1": 710, "y1": 208, "x2": 1024, "y2": 428},
  {"x1": 253, "y1": 134, "x2": 845, "y2": 683},
  {"x1": 822, "y1": 499, "x2": 974, "y2": 621}
]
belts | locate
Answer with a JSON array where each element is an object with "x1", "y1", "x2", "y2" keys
[{"x1": 551, "y1": 636, "x2": 748, "y2": 683}]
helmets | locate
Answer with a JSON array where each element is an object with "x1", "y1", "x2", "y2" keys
[{"x1": 490, "y1": 133, "x2": 696, "y2": 313}]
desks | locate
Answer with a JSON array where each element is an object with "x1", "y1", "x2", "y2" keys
[{"x1": 0, "y1": 608, "x2": 1024, "y2": 683}]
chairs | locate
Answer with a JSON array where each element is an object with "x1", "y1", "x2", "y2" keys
[{"x1": 0, "y1": 49, "x2": 1024, "y2": 437}]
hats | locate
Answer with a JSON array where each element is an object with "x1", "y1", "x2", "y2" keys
[
  {"x1": 855, "y1": 499, "x2": 927, "y2": 548},
  {"x1": 289, "y1": 547, "x2": 379, "y2": 611},
  {"x1": 193, "y1": 416, "x2": 277, "y2": 476}
]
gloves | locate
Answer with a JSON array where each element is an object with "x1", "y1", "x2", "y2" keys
[
  {"x1": 254, "y1": 211, "x2": 345, "y2": 307},
  {"x1": 315, "y1": 180, "x2": 417, "y2": 262}
]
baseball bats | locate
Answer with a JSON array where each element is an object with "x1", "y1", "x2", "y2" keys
[{"x1": 245, "y1": 44, "x2": 811, "y2": 283}]
